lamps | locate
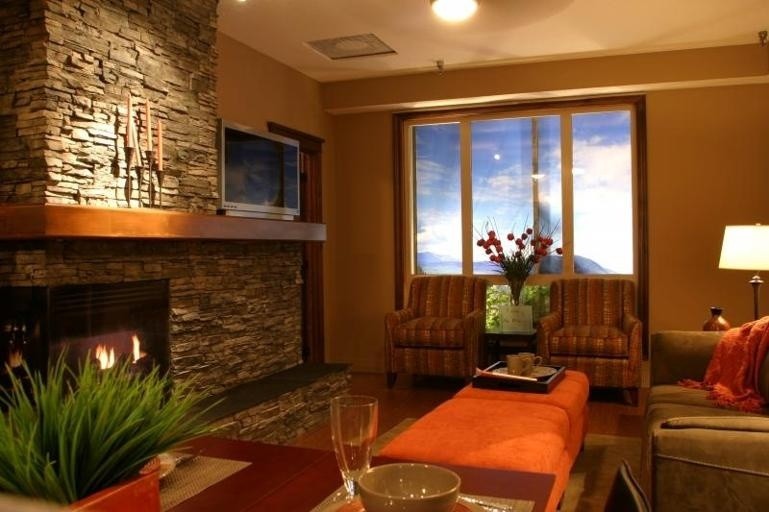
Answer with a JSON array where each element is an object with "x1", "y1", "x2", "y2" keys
[
  {"x1": 718, "y1": 223, "x2": 769, "y2": 320},
  {"x1": 429, "y1": 0, "x2": 480, "y2": 23}
]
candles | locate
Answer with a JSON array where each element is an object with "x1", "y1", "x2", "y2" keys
[
  {"x1": 132, "y1": 123, "x2": 141, "y2": 166},
  {"x1": 158, "y1": 120, "x2": 164, "y2": 170},
  {"x1": 145, "y1": 98, "x2": 153, "y2": 150},
  {"x1": 127, "y1": 94, "x2": 132, "y2": 146}
]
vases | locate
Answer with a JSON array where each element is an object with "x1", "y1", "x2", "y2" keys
[
  {"x1": 704, "y1": 307, "x2": 730, "y2": 331},
  {"x1": 500, "y1": 306, "x2": 533, "y2": 332}
]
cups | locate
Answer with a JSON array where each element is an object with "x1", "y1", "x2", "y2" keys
[
  {"x1": 329, "y1": 395, "x2": 378, "y2": 498},
  {"x1": 506, "y1": 352, "x2": 542, "y2": 376}
]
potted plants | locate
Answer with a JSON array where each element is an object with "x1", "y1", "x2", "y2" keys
[{"x1": 0, "y1": 348, "x2": 224, "y2": 512}]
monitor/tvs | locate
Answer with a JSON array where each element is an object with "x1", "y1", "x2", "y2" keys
[{"x1": 217, "y1": 118, "x2": 301, "y2": 221}]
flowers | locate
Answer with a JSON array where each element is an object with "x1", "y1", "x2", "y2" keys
[{"x1": 474, "y1": 215, "x2": 563, "y2": 306}]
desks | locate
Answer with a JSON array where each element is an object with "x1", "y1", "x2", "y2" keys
[{"x1": 483, "y1": 330, "x2": 538, "y2": 366}]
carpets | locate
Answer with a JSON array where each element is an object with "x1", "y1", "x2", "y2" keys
[{"x1": 370, "y1": 417, "x2": 642, "y2": 512}]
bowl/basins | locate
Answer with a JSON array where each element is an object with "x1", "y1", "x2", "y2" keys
[{"x1": 355, "y1": 463, "x2": 461, "y2": 511}]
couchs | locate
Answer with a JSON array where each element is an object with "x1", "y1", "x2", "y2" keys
[
  {"x1": 537, "y1": 278, "x2": 642, "y2": 407},
  {"x1": 642, "y1": 316, "x2": 769, "y2": 512},
  {"x1": 379, "y1": 370, "x2": 590, "y2": 512},
  {"x1": 385, "y1": 276, "x2": 485, "y2": 387}
]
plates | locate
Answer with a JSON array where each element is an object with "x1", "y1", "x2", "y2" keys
[{"x1": 492, "y1": 367, "x2": 558, "y2": 378}]
